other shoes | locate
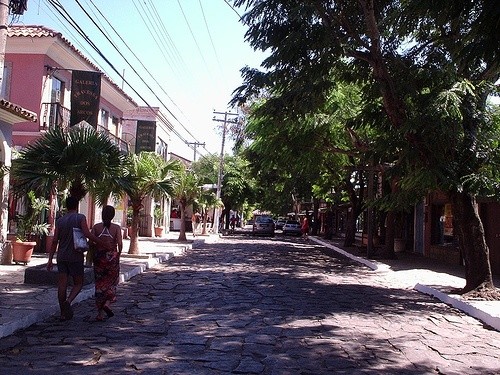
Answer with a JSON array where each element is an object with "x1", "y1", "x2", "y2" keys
[{"x1": 59, "y1": 300, "x2": 73, "y2": 322}]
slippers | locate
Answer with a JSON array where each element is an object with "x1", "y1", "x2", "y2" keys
[{"x1": 95, "y1": 307, "x2": 113, "y2": 321}]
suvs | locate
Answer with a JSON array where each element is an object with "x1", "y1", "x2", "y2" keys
[{"x1": 252, "y1": 216, "x2": 275, "y2": 238}]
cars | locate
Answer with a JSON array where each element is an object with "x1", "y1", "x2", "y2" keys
[
  {"x1": 273, "y1": 220, "x2": 287, "y2": 229},
  {"x1": 282, "y1": 220, "x2": 302, "y2": 236}
]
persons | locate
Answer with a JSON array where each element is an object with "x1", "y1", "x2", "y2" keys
[
  {"x1": 230, "y1": 215, "x2": 236, "y2": 230},
  {"x1": 192, "y1": 209, "x2": 201, "y2": 237},
  {"x1": 47, "y1": 197, "x2": 113, "y2": 319},
  {"x1": 172, "y1": 209, "x2": 177, "y2": 218},
  {"x1": 303, "y1": 215, "x2": 309, "y2": 241},
  {"x1": 86, "y1": 206, "x2": 122, "y2": 320}
]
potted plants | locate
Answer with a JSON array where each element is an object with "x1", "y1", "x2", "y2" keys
[
  {"x1": 126, "y1": 208, "x2": 142, "y2": 239},
  {"x1": 11, "y1": 192, "x2": 57, "y2": 261},
  {"x1": 153, "y1": 203, "x2": 164, "y2": 237},
  {"x1": 377, "y1": 190, "x2": 422, "y2": 252}
]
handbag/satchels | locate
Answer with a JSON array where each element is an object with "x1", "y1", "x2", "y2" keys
[{"x1": 72, "y1": 214, "x2": 90, "y2": 252}]
